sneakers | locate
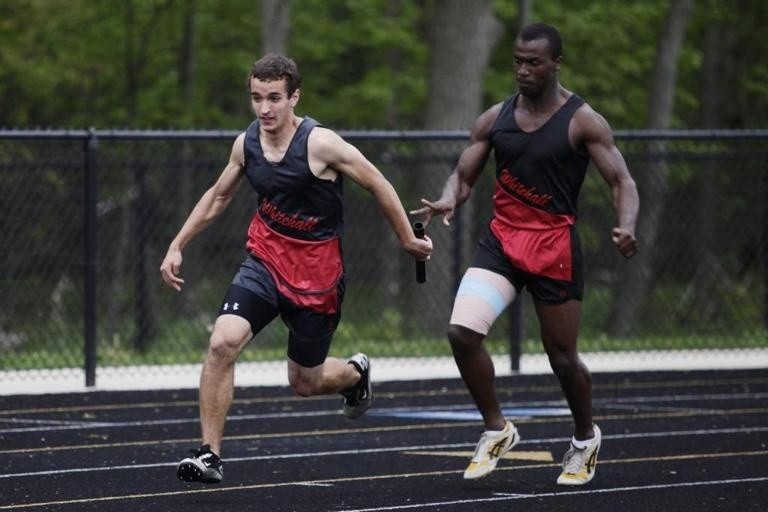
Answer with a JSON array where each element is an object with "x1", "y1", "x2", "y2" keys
[
  {"x1": 340, "y1": 352, "x2": 374, "y2": 421},
  {"x1": 175, "y1": 447, "x2": 227, "y2": 486},
  {"x1": 461, "y1": 419, "x2": 520, "y2": 480},
  {"x1": 555, "y1": 423, "x2": 604, "y2": 489}
]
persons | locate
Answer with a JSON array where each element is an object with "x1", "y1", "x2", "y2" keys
[
  {"x1": 158, "y1": 53, "x2": 434, "y2": 486},
  {"x1": 410, "y1": 24, "x2": 642, "y2": 488}
]
